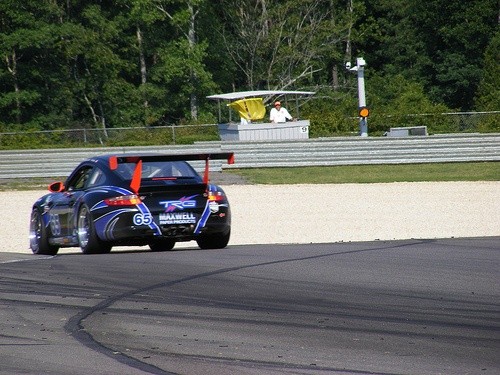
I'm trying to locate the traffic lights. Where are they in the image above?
[359,106,369,118]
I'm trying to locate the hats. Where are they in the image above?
[274,101,282,107]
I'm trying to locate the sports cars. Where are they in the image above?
[28,151,234,255]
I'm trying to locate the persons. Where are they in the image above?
[270,103,294,123]
